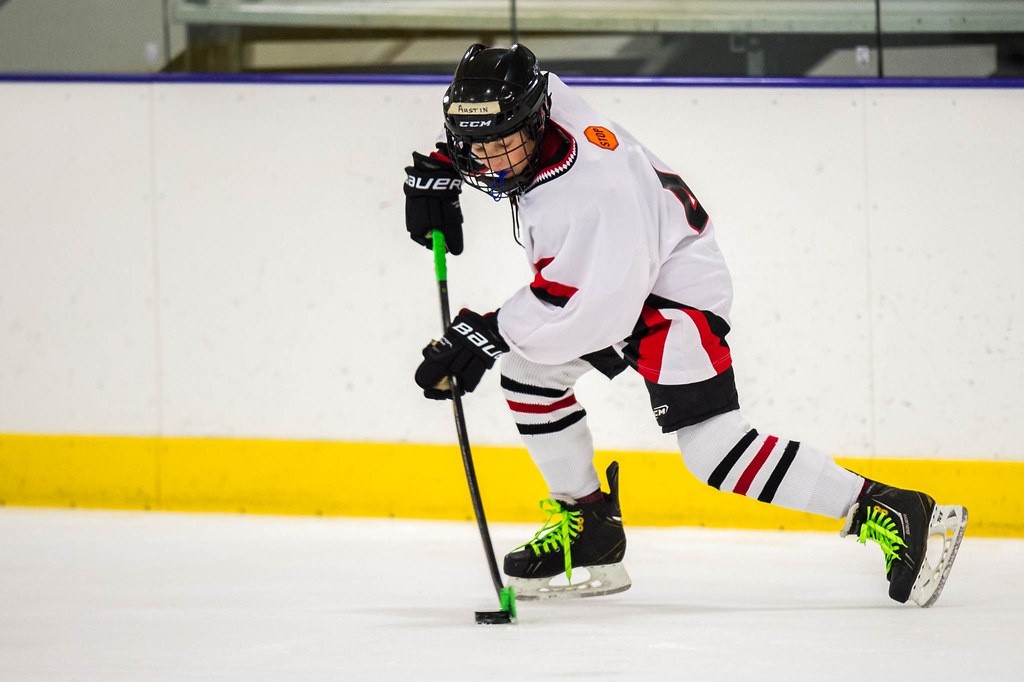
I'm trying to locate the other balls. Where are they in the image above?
[473,609,511,625]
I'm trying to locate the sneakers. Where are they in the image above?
[502,460,632,603]
[839,467,968,610]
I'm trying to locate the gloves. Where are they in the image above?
[402,150,464,256]
[414,307,511,401]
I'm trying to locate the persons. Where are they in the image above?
[402,41,969,627]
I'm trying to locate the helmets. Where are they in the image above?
[443,42,551,137]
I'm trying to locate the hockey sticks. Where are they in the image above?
[428,219,520,625]
